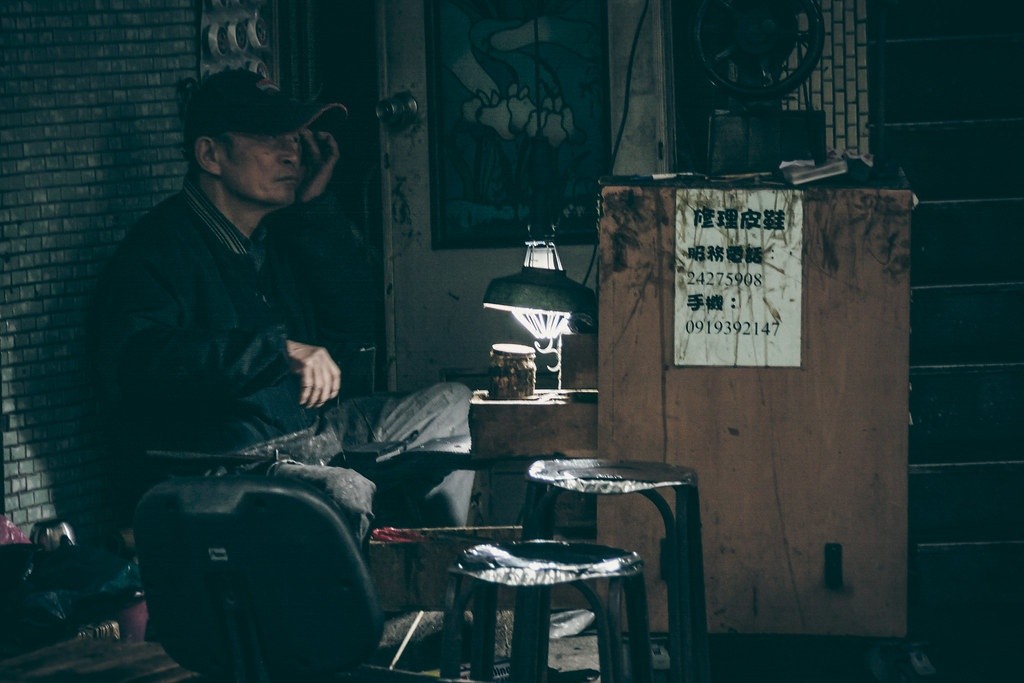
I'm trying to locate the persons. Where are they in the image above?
[91,71,596,642]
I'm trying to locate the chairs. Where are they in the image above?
[139,477,469,682]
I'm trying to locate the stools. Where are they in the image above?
[438,539,654,683]
[518,455,711,683]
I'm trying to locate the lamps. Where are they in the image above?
[480,236,597,372]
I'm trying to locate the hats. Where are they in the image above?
[185,69,349,138]
